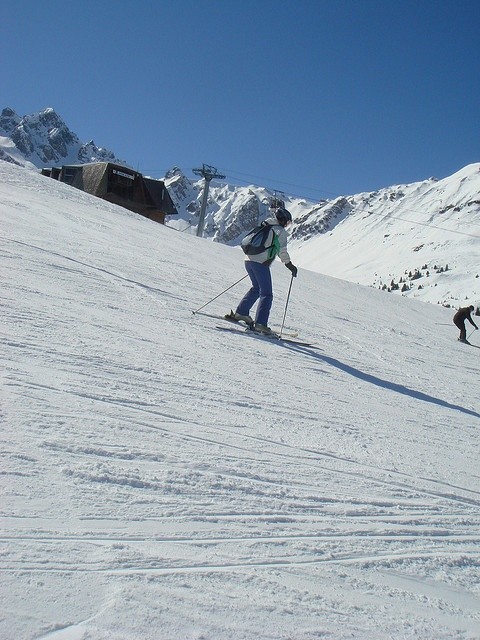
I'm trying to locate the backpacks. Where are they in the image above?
[241,221,284,254]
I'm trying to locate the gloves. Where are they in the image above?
[284,261,297,277]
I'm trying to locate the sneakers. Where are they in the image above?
[234,312,255,325]
[254,322,273,334]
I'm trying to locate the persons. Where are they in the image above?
[453,306,478,341]
[234,207,297,331]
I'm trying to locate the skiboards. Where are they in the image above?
[194,310,317,345]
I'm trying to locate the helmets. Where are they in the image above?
[276,206,292,227]
